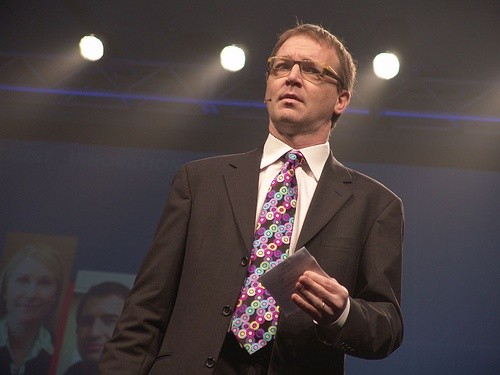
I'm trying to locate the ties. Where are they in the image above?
[231,150,305,354]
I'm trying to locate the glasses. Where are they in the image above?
[268,57,343,86]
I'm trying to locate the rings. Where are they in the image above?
[318,301,325,312]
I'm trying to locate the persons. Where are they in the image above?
[94,23,405,375]
[0,242,130,375]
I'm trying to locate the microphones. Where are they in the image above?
[264,99,272,101]
